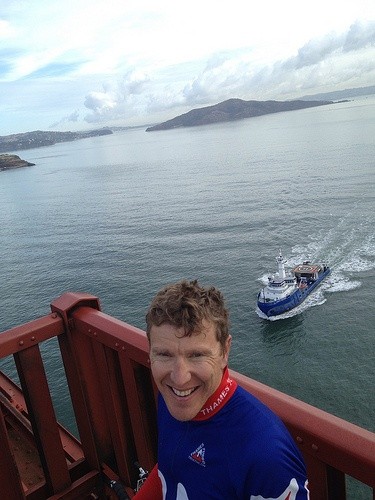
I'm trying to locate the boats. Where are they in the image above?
[257,248,330,318]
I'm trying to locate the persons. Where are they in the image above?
[130,279,311,500]
